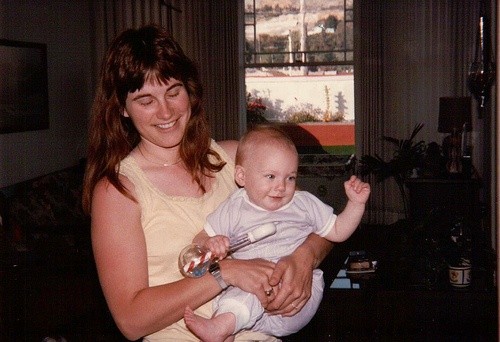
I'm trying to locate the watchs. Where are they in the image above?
[209,257,228,289]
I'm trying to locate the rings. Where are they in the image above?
[264,287,273,296]
[291,303,298,309]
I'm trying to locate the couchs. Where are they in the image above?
[0,159,125,342]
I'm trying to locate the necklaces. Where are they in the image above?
[137,145,181,166]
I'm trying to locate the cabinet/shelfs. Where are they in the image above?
[404,155,484,240]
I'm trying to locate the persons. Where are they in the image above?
[82,23,336,342]
[185,123,370,342]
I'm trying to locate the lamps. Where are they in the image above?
[437,95,473,174]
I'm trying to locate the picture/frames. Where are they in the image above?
[0,38,50,135]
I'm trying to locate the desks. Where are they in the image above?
[330,222,499,342]
